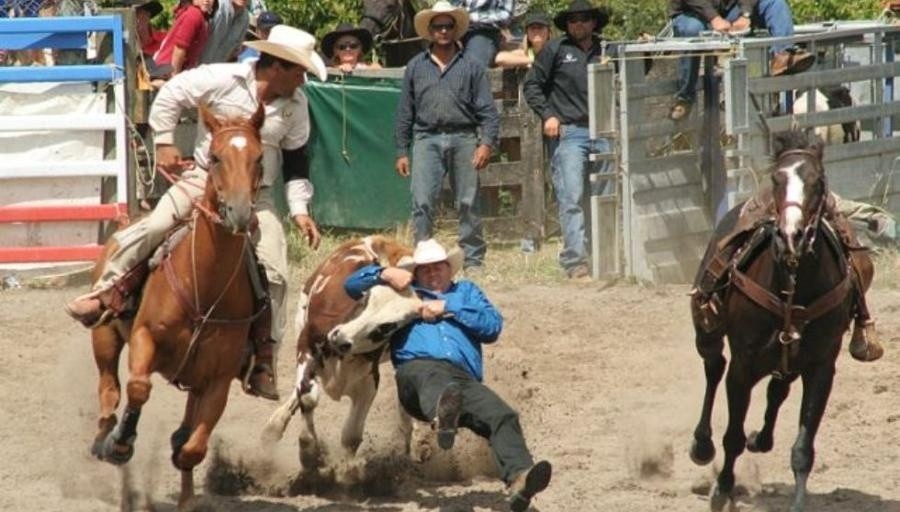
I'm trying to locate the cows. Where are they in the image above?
[260,219,460,490]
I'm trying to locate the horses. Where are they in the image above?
[87,100,268,512]
[688,122,859,512]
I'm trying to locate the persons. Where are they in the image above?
[199,1,248,65]
[663,2,819,122]
[341,236,554,512]
[442,2,523,78]
[62,22,327,401]
[0,1,65,66]
[494,12,555,216]
[524,2,657,285]
[238,10,283,62]
[134,0,219,80]
[392,1,501,276]
[321,21,383,79]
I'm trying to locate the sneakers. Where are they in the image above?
[510,460,550,511]
[437,383,461,450]
[671,100,692,121]
[770,47,815,77]
[247,364,278,402]
[63,298,112,327]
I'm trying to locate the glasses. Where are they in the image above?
[568,15,592,23]
[429,20,455,32]
[335,42,364,50]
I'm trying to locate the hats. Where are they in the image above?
[243,24,327,84]
[554,0,609,31]
[397,239,464,279]
[323,25,372,58]
[525,16,550,28]
[414,2,468,42]
[257,11,283,30]
